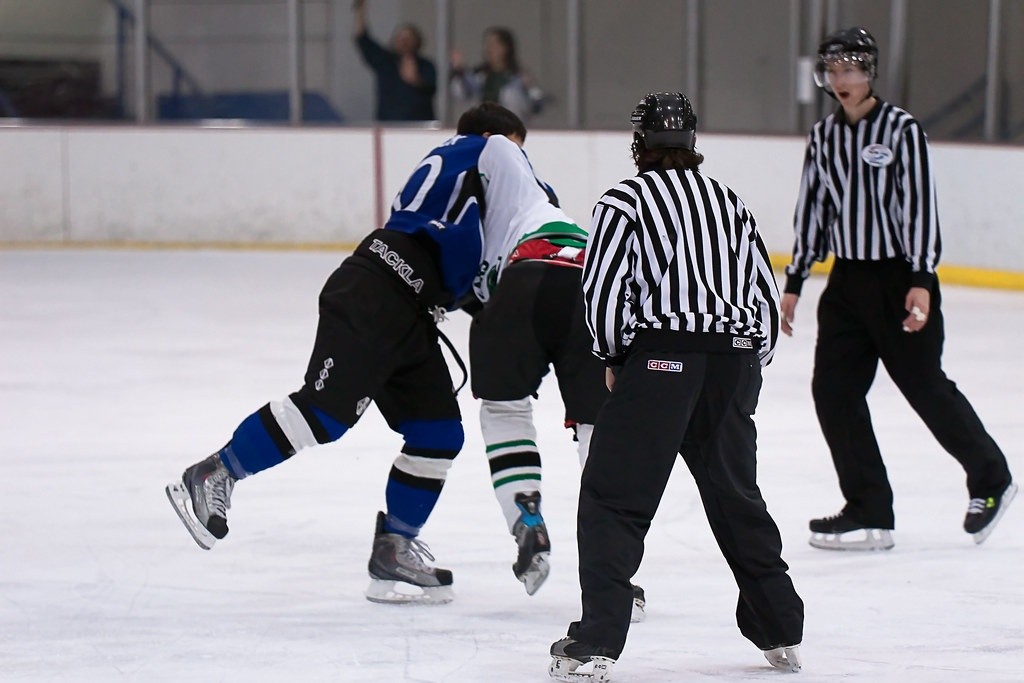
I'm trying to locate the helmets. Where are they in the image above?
[631,92,698,151]
[814,27,879,87]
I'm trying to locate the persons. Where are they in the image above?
[164,109,527,607]
[349,1,441,128]
[471,134,614,594]
[549,93,804,678]
[446,26,547,131]
[779,28,1019,549]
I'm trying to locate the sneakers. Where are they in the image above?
[631,586,652,623]
[166,452,237,551]
[548,637,617,683]
[762,641,802,673]
[512,490,551,595]
[808,511,896,551]
[963,477,1018,545]
[365,511,455,604]
